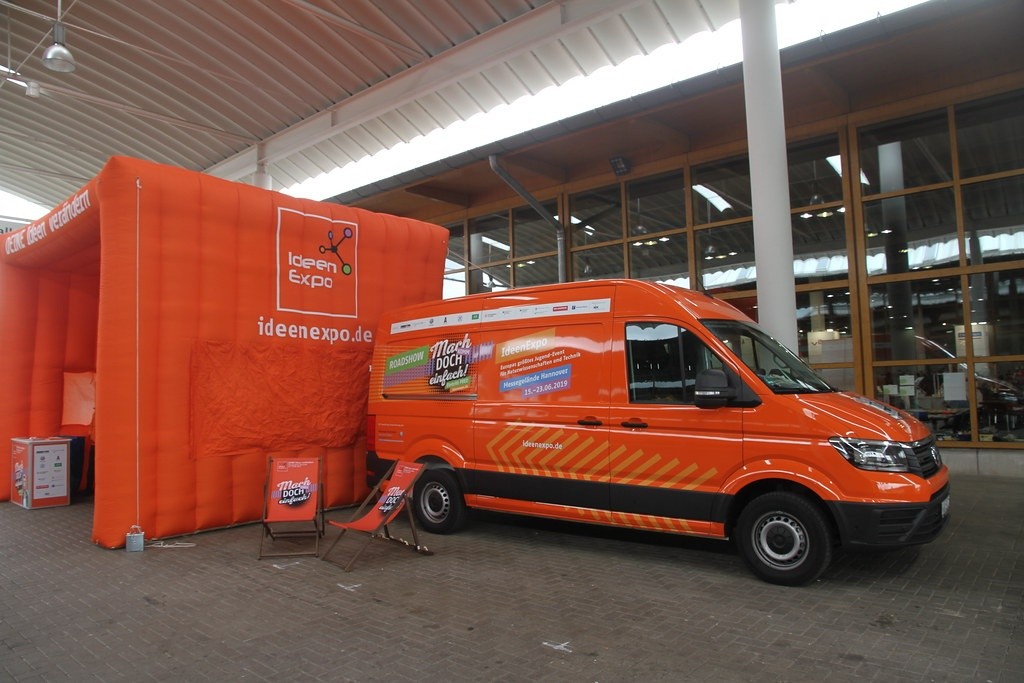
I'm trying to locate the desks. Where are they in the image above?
[979,399,1015,435]
[927,406,970,440]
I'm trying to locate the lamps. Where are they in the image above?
[483,243,496,289]
[609,156,632,177]
[800,160,838,220]
[24,81,42,99]
[583,233,594,274]
[705,227,740,261]
[41,0,78,73]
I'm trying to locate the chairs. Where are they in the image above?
[321,458,435,570]
[253,452,326,557]
[62,370,98,491]
[721,350,773,402]
[623,331,699,406]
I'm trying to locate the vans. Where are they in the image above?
[838,333,1024,440]
[364,279,950,585]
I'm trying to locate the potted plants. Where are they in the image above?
[977,430,995,442]
[941,412,972,441]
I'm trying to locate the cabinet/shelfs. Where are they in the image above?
[10,438,73,510]
